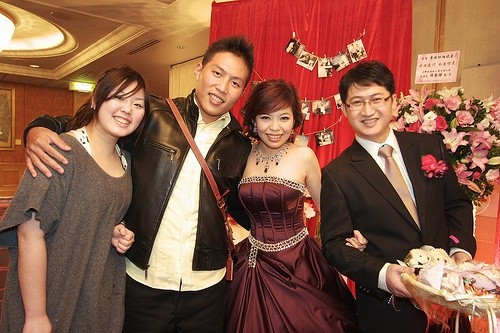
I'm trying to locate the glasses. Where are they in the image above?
[344,94,392,110]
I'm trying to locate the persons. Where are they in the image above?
[23,36,306,333]
[224,79,368,333]
[320,60,477,333]
[0,64,150,333]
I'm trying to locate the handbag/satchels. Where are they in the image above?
[225,222,235,281]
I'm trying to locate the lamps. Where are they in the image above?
[0,10,16,51]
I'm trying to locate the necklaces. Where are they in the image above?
[254,141,290,173]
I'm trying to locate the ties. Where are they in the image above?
[378,144,421,232]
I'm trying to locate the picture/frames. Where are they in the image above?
[0,87,16,150]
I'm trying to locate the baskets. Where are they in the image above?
[400,262,500,318]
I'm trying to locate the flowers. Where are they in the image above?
[421,153,449,180]
[391,83,497,206]
